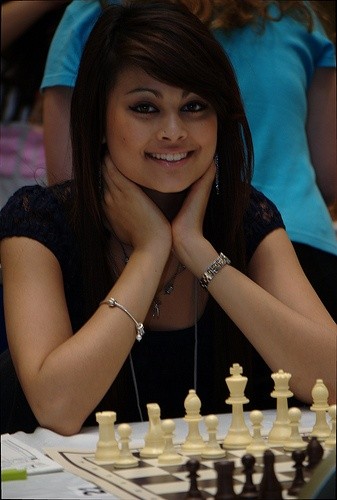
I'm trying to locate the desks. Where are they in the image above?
[1,411,330,500]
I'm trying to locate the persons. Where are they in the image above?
[0,0,337,434]
[0,0,337,321]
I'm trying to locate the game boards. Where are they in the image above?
[46,360,337,500]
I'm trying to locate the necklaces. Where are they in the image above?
[118,240,188,319]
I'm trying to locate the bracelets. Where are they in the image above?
[198,252,231,288]
[100,298,146,341]
